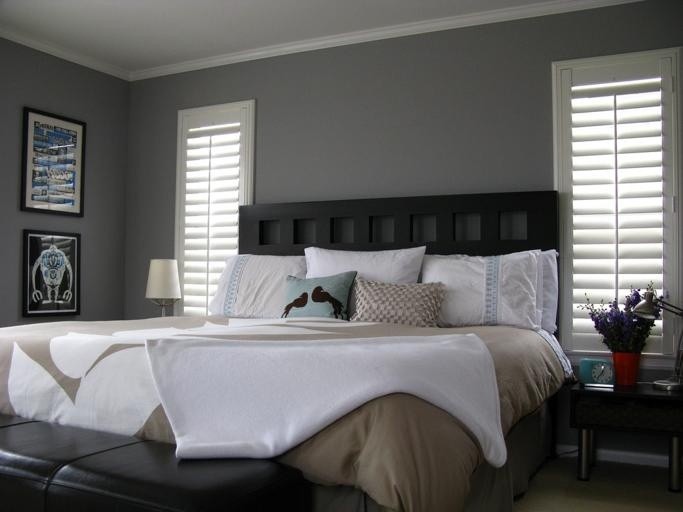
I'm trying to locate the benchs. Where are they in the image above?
[0,414,305,512]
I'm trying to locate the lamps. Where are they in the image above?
[633,292,683,391]
[145,259,182,317]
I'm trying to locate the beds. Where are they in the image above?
[0,191,558,512]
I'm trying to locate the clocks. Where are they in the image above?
[592,363,613,384]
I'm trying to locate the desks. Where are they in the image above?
[569,381,683,493]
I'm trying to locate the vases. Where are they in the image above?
[612,352,641,389]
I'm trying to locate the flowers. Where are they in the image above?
[586,282,664,353]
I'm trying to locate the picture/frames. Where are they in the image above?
[21,106,86,218]
[23,229,80,317]
